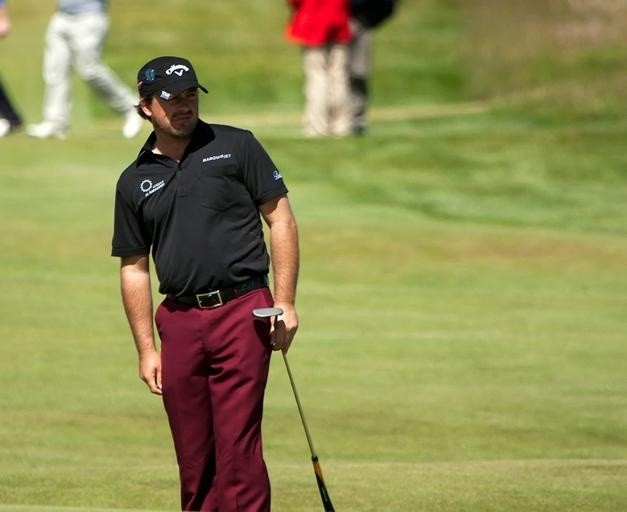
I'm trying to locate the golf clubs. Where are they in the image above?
[252,308,335,512]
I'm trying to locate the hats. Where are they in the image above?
[133,55,209,103]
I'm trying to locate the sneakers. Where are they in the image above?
[22,121,67,140]
[121,98,145,140]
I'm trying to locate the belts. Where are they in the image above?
[164,273,269,312]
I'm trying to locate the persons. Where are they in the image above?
[283,0,395,141]
[27,0,145,141]
[109,57,299,511]
[0,0,22,138]
